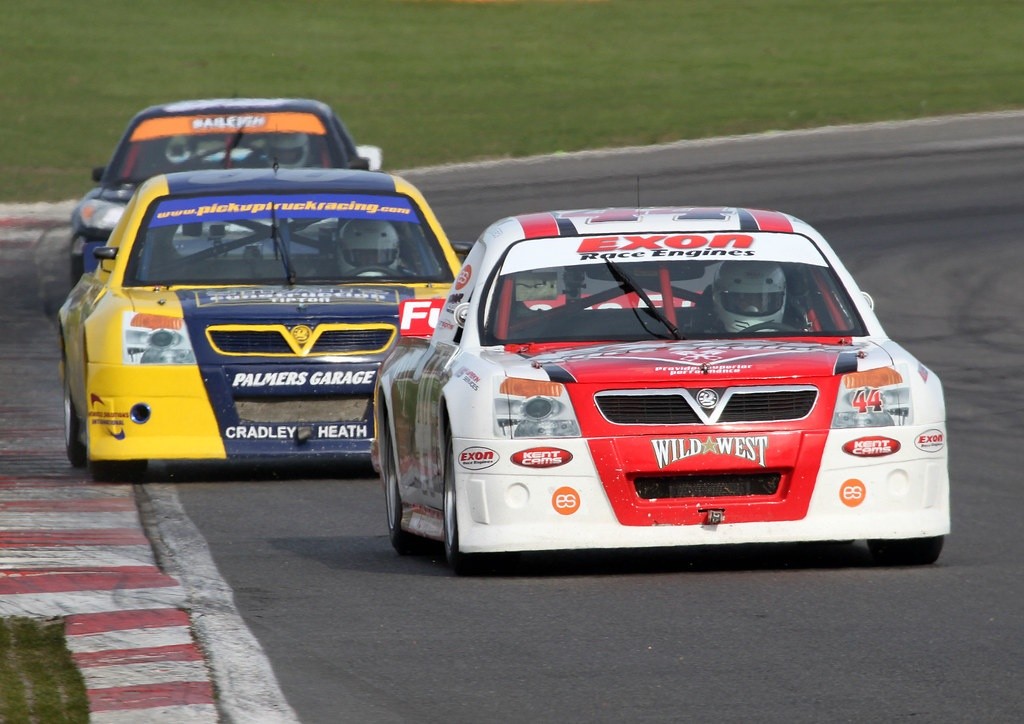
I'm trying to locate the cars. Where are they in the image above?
[57,166,471,481]
[374,206,952,577]
[70,95,370,282]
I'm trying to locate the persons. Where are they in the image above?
[336,219,417,277]
[267,134,314,169]
[709,259,788,337]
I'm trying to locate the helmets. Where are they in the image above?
[338,220,400,277]
[712,261,786,333]
[267,132,309,169]
